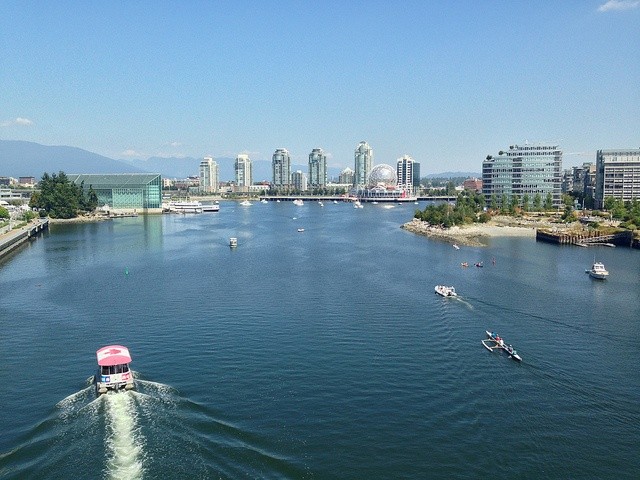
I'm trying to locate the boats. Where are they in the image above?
[582,251,609,281]
[479,329,523,363]
[434,284,456,298]
[229,238,238,248]
[92,344,137,397]
